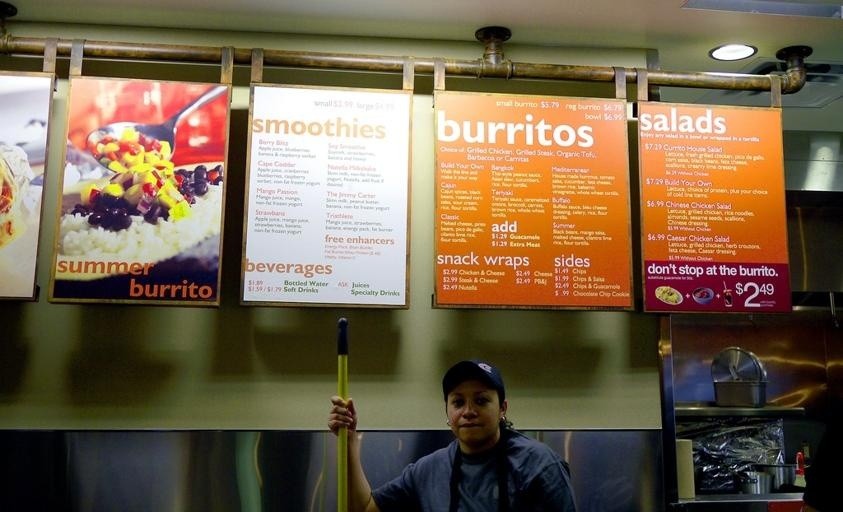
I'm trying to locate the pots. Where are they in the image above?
[714,380,768,409]
[736,459,797,496]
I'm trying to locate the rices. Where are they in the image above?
[55,181,223,280]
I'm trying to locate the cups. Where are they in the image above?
[724,290,733,306]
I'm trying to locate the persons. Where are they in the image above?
[327,359,578,509]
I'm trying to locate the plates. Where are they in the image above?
[654,286,683,305]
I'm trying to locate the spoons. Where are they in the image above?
[86,81,228,176]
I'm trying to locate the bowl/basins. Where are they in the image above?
[692,287,714,305]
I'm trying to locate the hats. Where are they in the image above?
[442,359,505,402]
